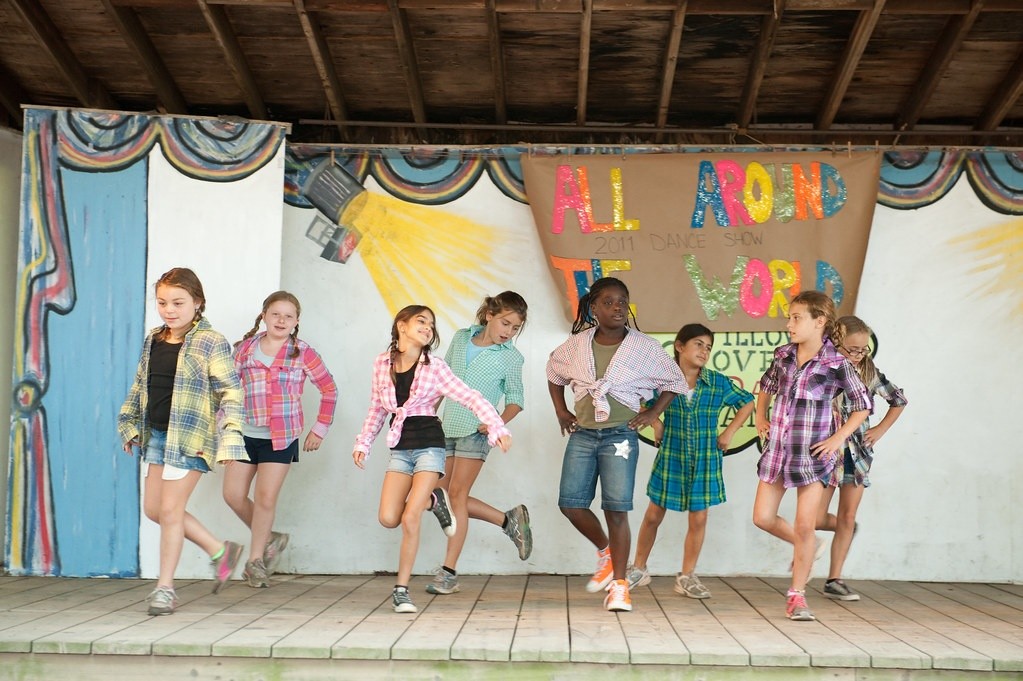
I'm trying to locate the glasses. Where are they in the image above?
[840,344,873,358]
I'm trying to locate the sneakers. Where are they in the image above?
[145,585,182,615]
[823,577,860,602]
[586,545,615,594]
[788,537,827,570]
[429,486,457,537]
[622,564,651,591]
[673,571,713,598]
[240,557,270,588]
[210,539,245,596]
[603,579,633,611]
[502,504,533,560]
[262,530,290,569]
[425,564,460,595]
[783,592,816,621]
[391,587,417,614]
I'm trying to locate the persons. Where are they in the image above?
[349,304,512,612]
[427,289,532,595]
[222,290,339,588]
[545,278,689,613]
[625,324,755,599]
[116,267,254,616]
[753,289,873,620]
[813,315,908,600]
